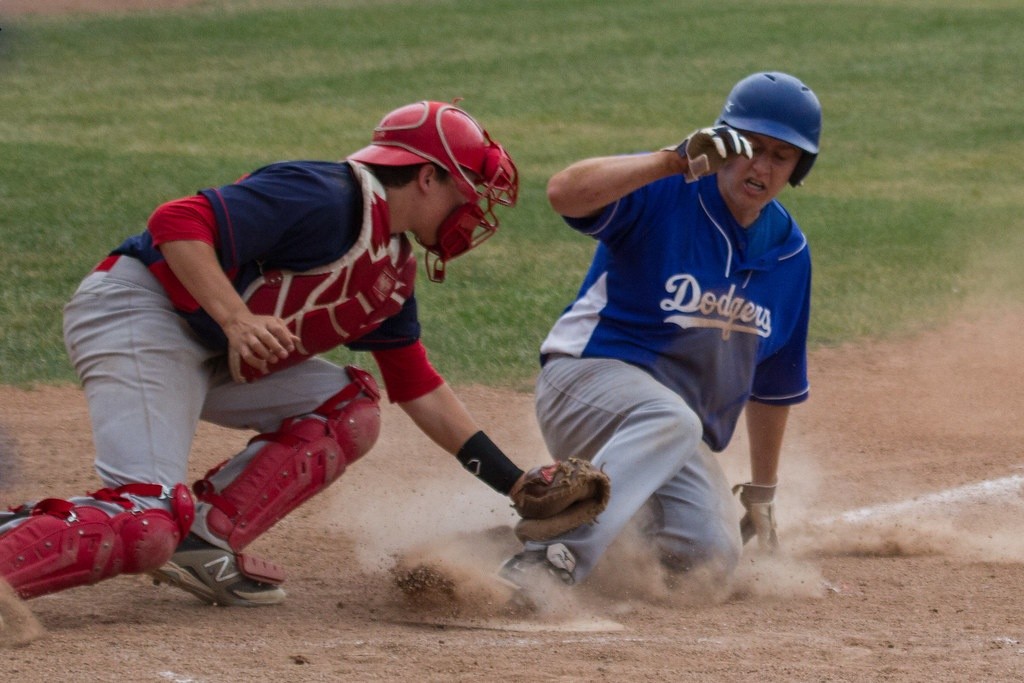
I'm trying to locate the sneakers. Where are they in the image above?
[494,542,578,597]
[144,506,286,604]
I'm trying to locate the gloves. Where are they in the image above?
[731,478,778,550]
[660,126,754,184]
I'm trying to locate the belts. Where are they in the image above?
[89,256,119,272]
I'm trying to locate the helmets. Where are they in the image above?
[346,100,487,174]
[713,71,820,187]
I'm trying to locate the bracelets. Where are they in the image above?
[457,431,525,499]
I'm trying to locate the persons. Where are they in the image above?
[1,99,610,603]
[491,71,825,592]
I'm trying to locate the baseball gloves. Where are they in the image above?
[510,456,613,544]
